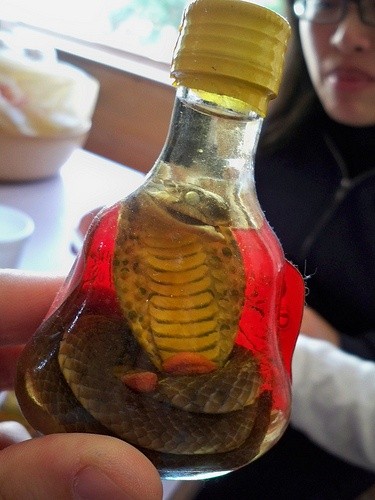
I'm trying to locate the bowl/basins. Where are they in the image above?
[0,123,92,183]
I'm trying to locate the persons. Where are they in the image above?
[79,0,375,364]
[0,269,375,500]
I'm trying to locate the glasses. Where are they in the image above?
[292,0,375,27]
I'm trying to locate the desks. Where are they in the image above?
[0,149,204,500]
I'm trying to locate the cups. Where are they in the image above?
[0,206,35,270]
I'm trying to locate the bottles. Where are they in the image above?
[14,0,307,478]
